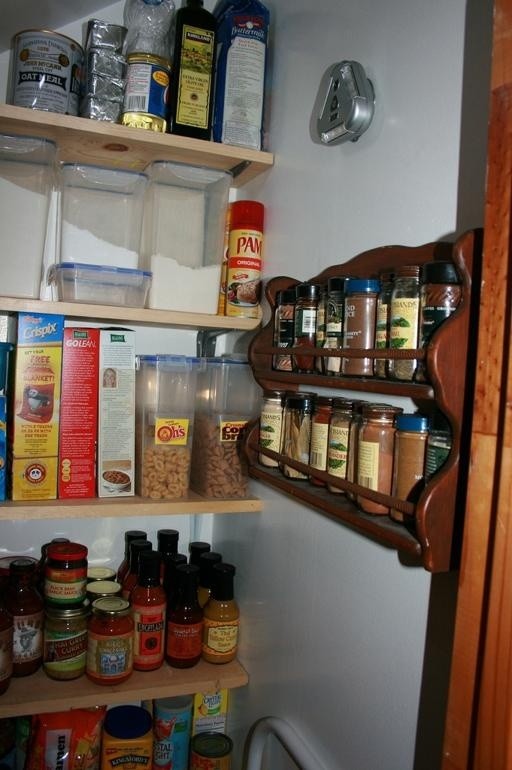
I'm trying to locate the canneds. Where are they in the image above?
[188,732,233,770]
[261,387,452,516]
[272,265,461,382]
[0,538,134,686]
[119,53,172,135]
[8,29,83,116]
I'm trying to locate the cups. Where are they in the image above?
[134,353,257,502]
[60,158,150,317]
[143,156,226,312]
[0,128,56,300]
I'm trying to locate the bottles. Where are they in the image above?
[261,386,459,523]
[0,559,46,693]
[120,528,241,695]
[123,0,270,152]
[215,198,267,318]
[268,259,460,381]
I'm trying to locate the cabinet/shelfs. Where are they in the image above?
[1,94,263,720]
[240,232,487,572]
